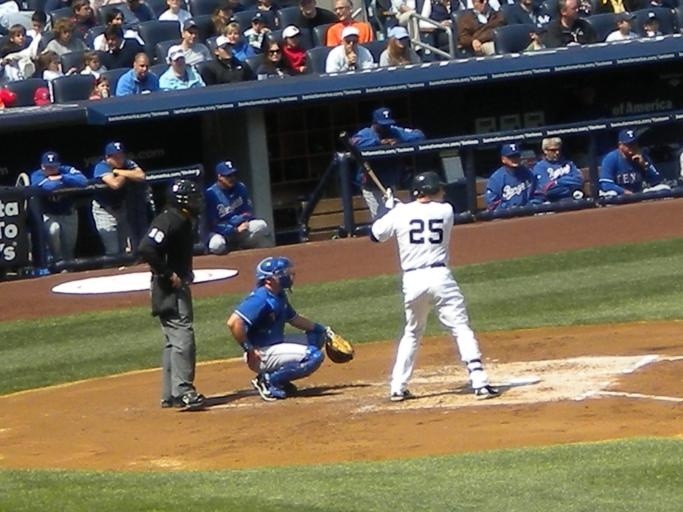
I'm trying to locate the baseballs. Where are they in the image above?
[573,190,584,200]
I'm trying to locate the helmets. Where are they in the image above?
[170,179,205,217]
[408,171,448,197]
[256,257,296,293]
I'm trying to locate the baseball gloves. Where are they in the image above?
[326,325,354,363]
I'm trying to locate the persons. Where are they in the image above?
[204,160,268,255]
[1,1,683,108]
[367,170,500,402]
[92,142,145,269]
[137,179,205,411]
[226,256,335,402]
[349,109,426,237]
[484,141,538,212]
[598,129,662,204]
[31,152,89,274]
[529,137,585,205]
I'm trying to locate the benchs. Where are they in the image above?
[293,161,608,245]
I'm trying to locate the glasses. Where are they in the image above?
[170,50,183,56]
[270,48,281,56]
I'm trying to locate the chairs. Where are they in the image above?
[0,0,683,114]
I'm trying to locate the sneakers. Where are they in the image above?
[390,387,414,401]
[160,391,205,412]
[474,386,500,400]
[251,373,278,401]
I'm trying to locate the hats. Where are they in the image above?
[105,141,126,155]
[214,35,236,49]
[616,128,640,144]
[34,88,50,106]
[387,26,410,40]
[500,143,523,158]
[251,11,265,23]
[614,11,658,24]
[41,151,61,167]
[216,160,239,177]
[283,25,300,40]
[168,45,185,62]
[372,107,396,126]
[181,18,198,31]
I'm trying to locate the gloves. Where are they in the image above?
[381,187,402,209]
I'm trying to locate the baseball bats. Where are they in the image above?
[340,132,386,194]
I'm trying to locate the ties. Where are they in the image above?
[342,26,360,39]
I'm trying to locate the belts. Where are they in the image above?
[404,262,445,272]
